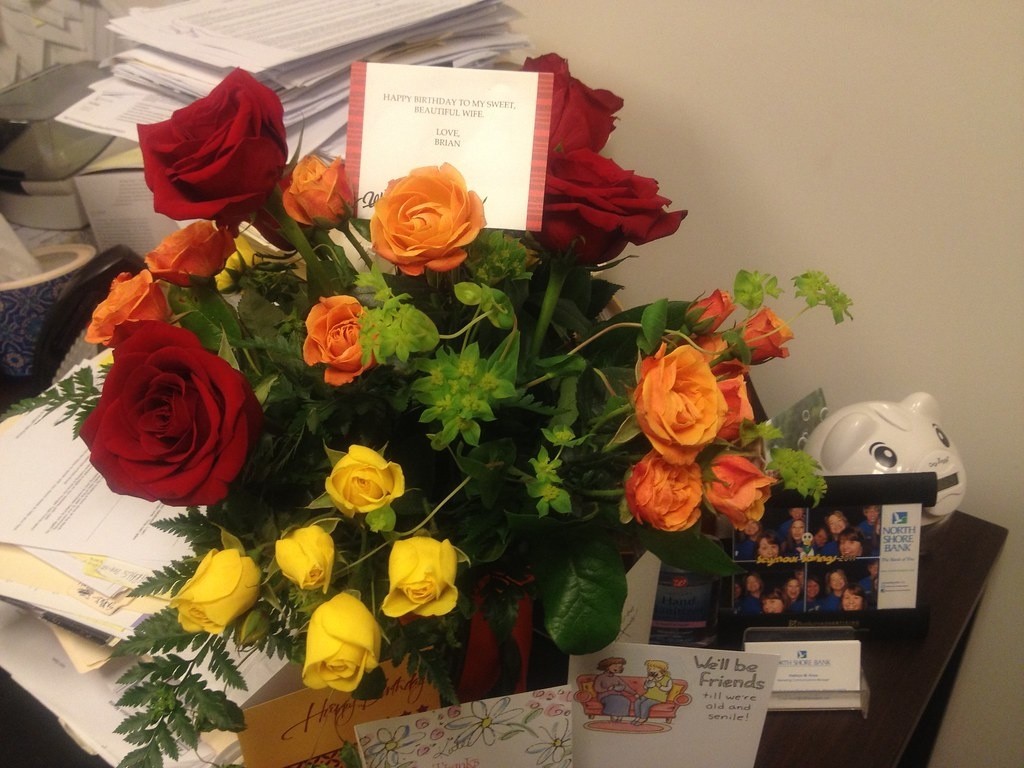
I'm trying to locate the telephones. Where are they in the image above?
[32,243,150,389]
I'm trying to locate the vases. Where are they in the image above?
[369,563,535,707]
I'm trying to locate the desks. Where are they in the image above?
[0,240,1012,768]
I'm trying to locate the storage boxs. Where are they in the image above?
[73,137,193,260]
[234,644,441,768]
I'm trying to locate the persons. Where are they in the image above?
[733,504,881,612]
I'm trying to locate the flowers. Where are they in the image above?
[0,51,861,768]
